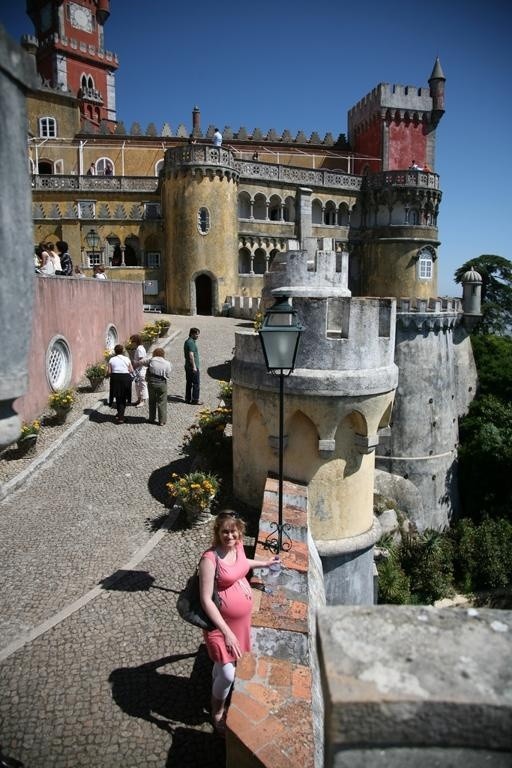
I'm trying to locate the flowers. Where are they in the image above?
[155,317,171,327]
[164,471,221,509]
[19,419,43,436]
[141,321,161,341]
[218,380,233,397]
[103,348,114,360]
[84,361,108,378]
[194,405,231,428]
[177,424,210,460]
[48,388,78,410]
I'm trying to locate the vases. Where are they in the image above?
[219,397,233,406]
[88,375,107,392]
[160,325,170,338]
[181,496,215,526]
[189,454,215,474]
[52,407,76,425]
[16,435,38,460]
[206,425,226,438]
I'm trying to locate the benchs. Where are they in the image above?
[143,303,162,314]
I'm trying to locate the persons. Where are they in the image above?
[188,133,196,144]
[138,348,171,426]
[130,334,148,408]
[184,328,204,405]
[107,344,133,424]
[105,163,113,176]
[198,516,284,739]
[33,240,107,281]
[90,163,95,176]
[212,129,222,147]
[408,159,432,172]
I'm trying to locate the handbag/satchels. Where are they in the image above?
[177,576,220,632]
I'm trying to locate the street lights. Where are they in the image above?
[85,229,101,278]
[258,292,309,553]
